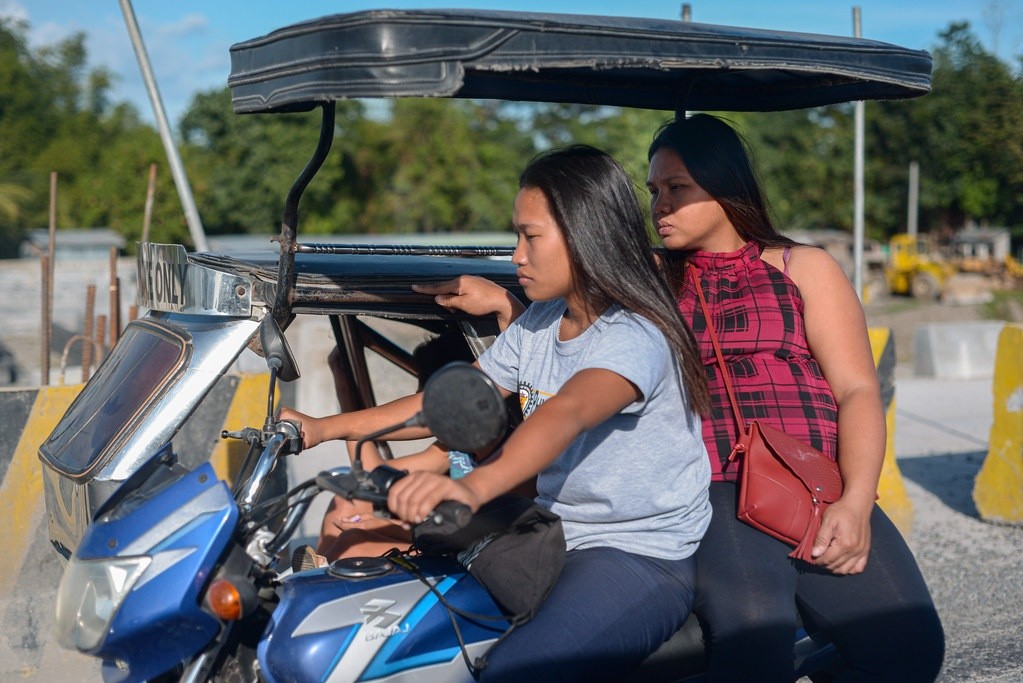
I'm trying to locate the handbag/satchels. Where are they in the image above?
[736,420,880,565]
[380,487,568,673]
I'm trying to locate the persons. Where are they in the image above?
[410,113,946,683]
[290,275,528,572]
[278,145,715,683]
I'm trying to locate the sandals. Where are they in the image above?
[291,544,330,573]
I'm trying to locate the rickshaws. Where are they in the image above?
[37,6,940,681]
